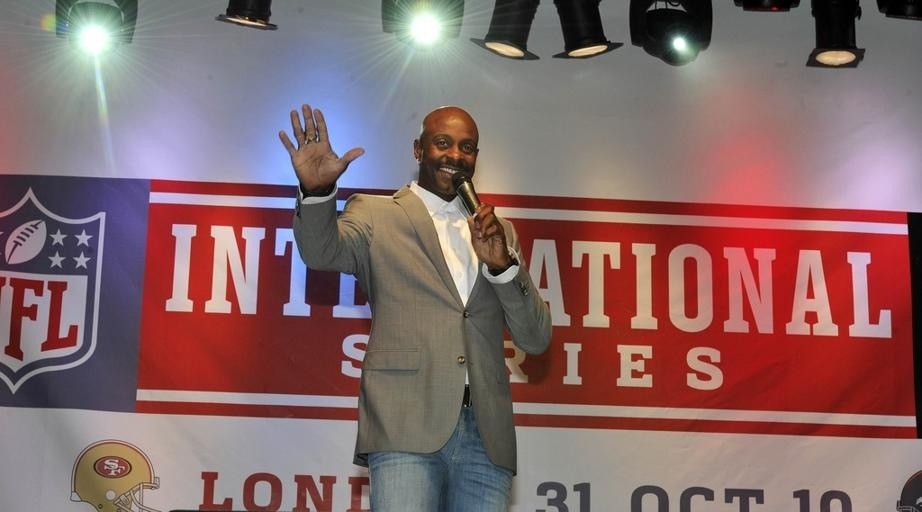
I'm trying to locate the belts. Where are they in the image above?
[462,384,472,406]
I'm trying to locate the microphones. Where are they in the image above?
[452,172,480,217]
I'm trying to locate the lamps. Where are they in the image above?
[55,0,137,45]
[381,0,464,49]
[469,0,542,60]
[628,1,711,66]
[550,0,623,59]
[805,0,865,67]
[216,0,278,30]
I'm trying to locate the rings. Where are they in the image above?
[305,131,317,141]
[297,134,304,138]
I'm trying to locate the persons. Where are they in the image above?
[280,103,552,512]
